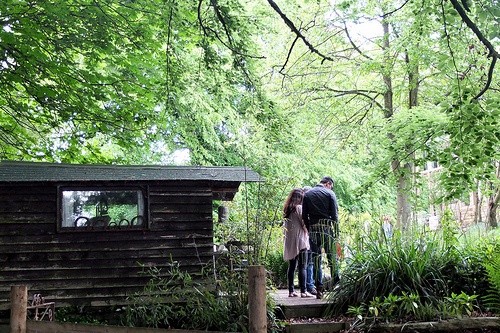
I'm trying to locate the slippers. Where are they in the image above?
[302,293,313,298]
[289,293,298,297]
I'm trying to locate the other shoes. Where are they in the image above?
[310,287,324,299]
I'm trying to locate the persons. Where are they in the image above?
[382,217,393,236]
[344,239,357,264]
[301,185,317,297]
[301,176,341,300]
[415,233,429,251]
[281,188,314,298]
[335,242,343,268]
